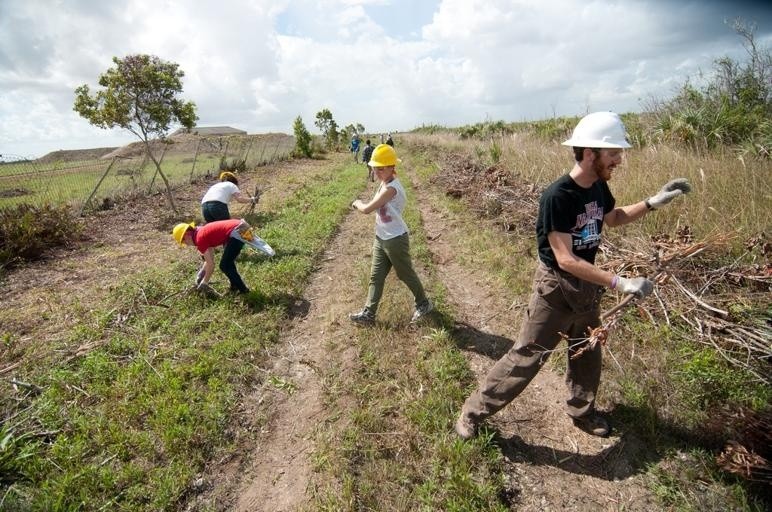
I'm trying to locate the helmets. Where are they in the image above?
[173,222,195,247]
[220,172,236,180]
[368,144,402,167]
[560,112,633,148]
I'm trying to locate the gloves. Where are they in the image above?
[196,270,208,293]
[612,274,654,299]
[649,178,692,208]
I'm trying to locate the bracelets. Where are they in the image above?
[644,198,657,212]
[252,196,257,204]
[197,269,206,279]
[611,275,617,289]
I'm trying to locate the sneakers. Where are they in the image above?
[456,412,478,439]
[412,300,433,321]
[577,414,609,435]
[349,312,375,322]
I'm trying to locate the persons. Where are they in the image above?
[347,133,393,182]
[201,172,259,225]
[456,111,691,444]
[173,219,254,294]
[349,144,433,325]
